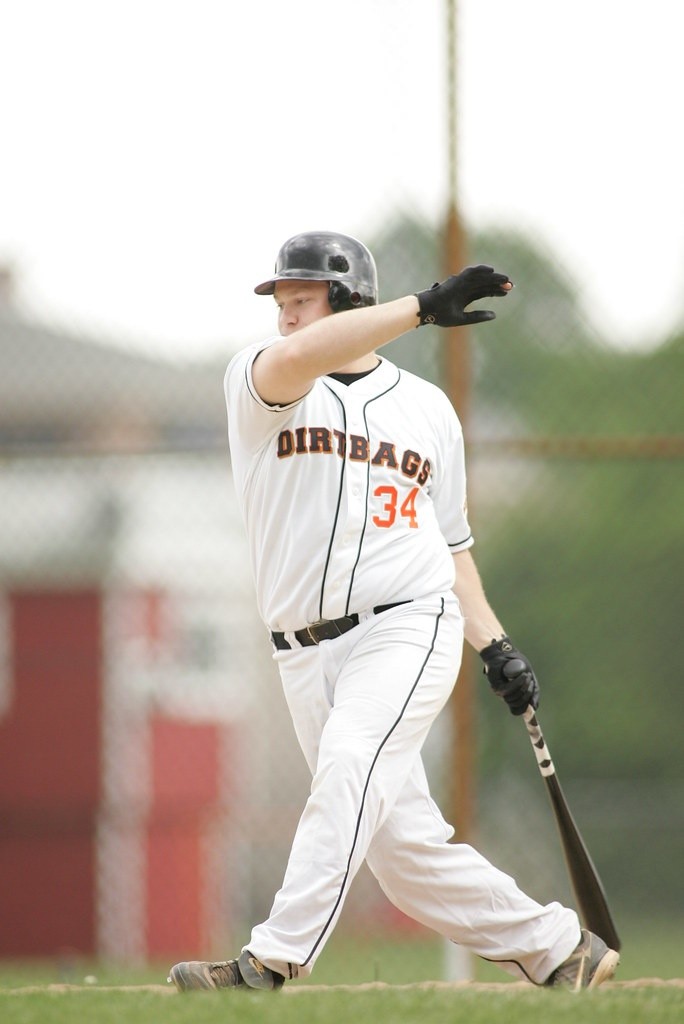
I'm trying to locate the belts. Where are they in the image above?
[272,600,412,650]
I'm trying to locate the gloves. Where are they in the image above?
[415,264,514,328]
[480,634,539,715]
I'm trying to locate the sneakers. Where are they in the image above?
[545,929,620,996]
[170,950,285,995]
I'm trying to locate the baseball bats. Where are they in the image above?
[514,695,624,958]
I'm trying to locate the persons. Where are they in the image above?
[167,233,619,992]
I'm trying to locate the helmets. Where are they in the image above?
[254,231,378,314]
